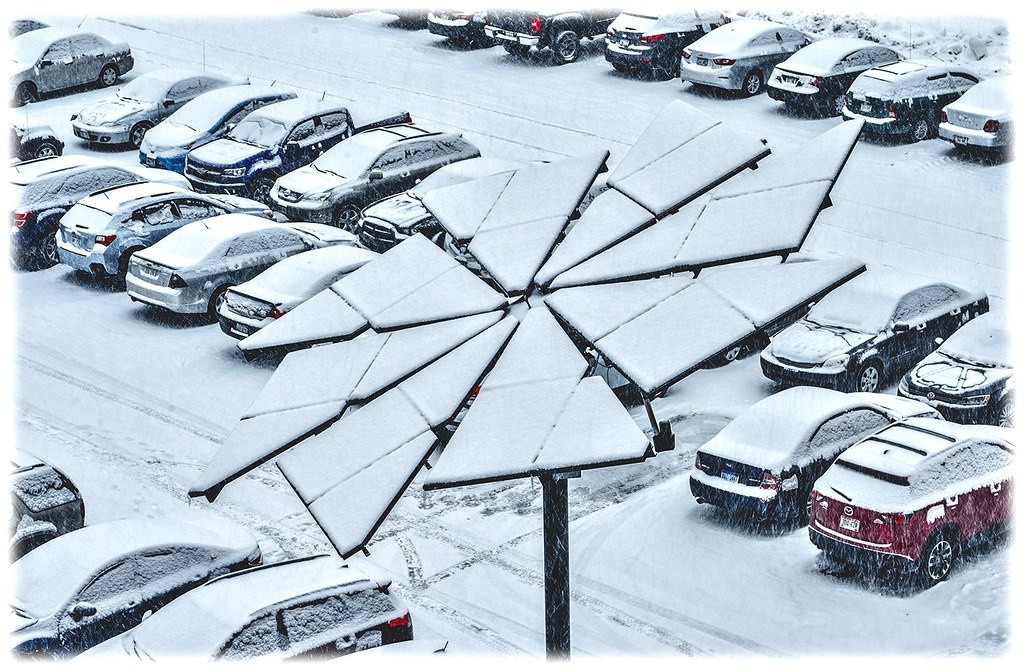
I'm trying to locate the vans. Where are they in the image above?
[67,552,415,664]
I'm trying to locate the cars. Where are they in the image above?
[69,64,251,149]
[679,18,825,97]
[269,121,557,295]
[938,73,1014,163]
[11,511,264,663]
[125,212,359,324]
[10,447,85,566]
[138,83,301,176]
[766,38,909,118]
[298,7,623,66]
[689,384,948,530]
[759,267,990,394]
[217,243,383,360]
[10,119,64,163]
[9,24,136,108]
[9,153,196,274]
[896,310,1018,428]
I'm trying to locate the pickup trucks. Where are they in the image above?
[183,93,414,211]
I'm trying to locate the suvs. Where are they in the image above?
[602,8,731,81]
[52,178,279,292]
[841,59,986,144]
[806,417,1015,590]
